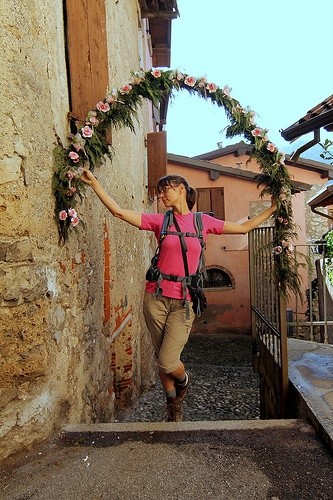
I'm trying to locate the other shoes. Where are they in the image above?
[175,370,192,401]
[167,398,183,422]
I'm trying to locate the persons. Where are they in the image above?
[79,167,279,421]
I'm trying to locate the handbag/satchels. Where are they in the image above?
[188,285,208,315]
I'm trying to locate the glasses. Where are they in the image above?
[157,181,184,193]
